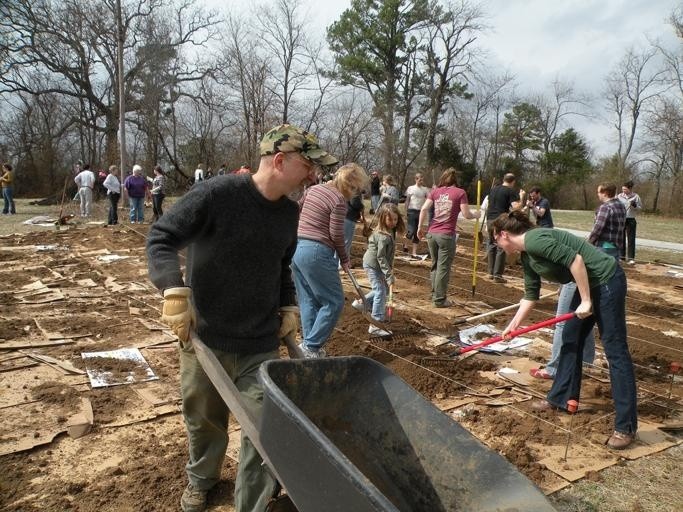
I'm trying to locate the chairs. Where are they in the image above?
[351,300,363,312]
[435,301,450,307]
[531,400,556,410]
[410,255,422,260]
[298,342,318,359]
[368,325,393,336]
[493,276,507,283]
[606,430,633,449]
[629,259,635,265]
[403,243,408,253]
[529,368,553,379]
[180,483,208,512]
[317,348,326,358]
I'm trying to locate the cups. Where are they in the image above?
[493,235,500,245]
[285,152,321,177]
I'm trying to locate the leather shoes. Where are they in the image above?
[161,286,196,342]
[631,201,636,208]
[276,306,298,353]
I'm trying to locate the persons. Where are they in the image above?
[0,163,17,216]
[101,164,121,226]
[333,195,365,270]
[616,180,641,263]
[194,160,250,184]
[291,162,368,360]
[417,167,481,308]
[528,277,596,380]
[144,165,166,220]
[487,173,526,282]
[144,123,340,512]
[403,172,432,260]
[351,203,406,337]
[377,174,400,241]
[529,187,553,228]
[488,208,638,449]
[123,163,147,225]
[586,182,627,262]
[370,171,380,213]
[74,163,96,217]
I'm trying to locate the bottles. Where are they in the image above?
[259,124,338,166]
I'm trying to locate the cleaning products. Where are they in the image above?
[178,313,558,511]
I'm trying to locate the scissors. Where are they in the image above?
[477,177,496,244]
[360,192,372,237]
[347,266,393,336]
